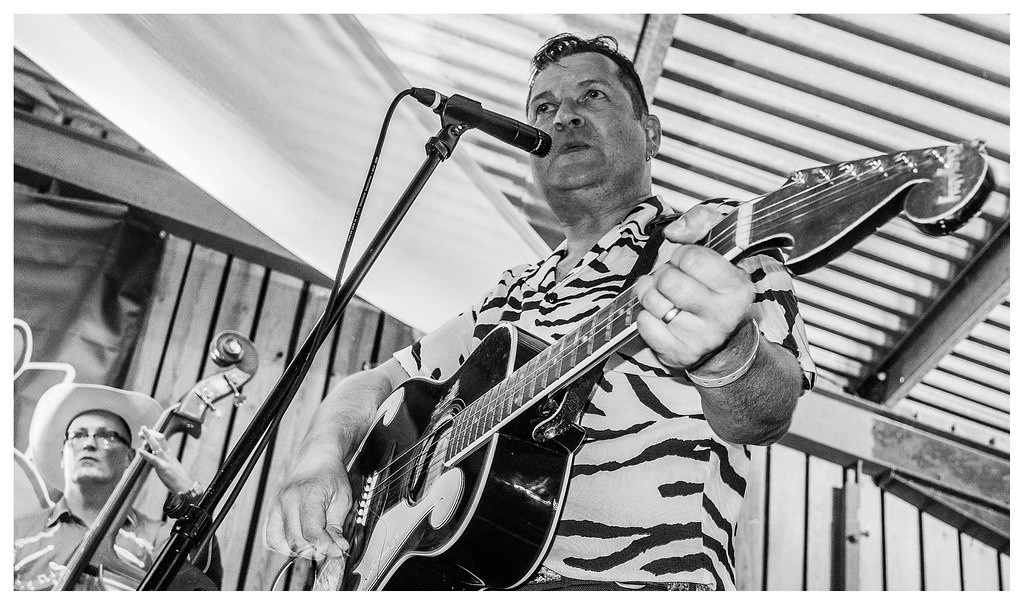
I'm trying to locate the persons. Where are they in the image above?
[264,32,818,591]
[12,383,223,591]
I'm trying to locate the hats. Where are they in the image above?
[29,383,166,511]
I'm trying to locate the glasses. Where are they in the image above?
[64,430,131,449]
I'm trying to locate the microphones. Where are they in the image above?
[411,88,552,158]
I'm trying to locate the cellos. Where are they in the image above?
[50,328,257,590]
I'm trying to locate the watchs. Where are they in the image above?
[182,481,203,501]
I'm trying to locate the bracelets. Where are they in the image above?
[684,316,760,388]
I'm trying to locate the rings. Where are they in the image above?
[152,448,162,455]
[662,305,682,324]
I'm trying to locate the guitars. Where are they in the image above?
[266,137,995,590]
